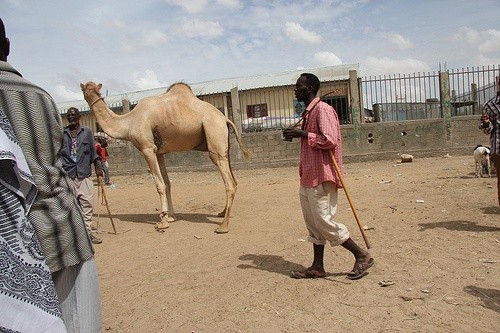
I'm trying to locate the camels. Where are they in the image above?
[79,80,252,233]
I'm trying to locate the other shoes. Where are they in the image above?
[89,234,102,244]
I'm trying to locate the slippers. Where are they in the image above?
[346,258,374,279]
[291,268,323,279]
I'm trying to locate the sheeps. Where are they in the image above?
[472,144,492,178]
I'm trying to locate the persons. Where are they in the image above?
[478,78,500,207]
[281,73,375,280]
[61,107,112,244]
[0,17,103,333]
